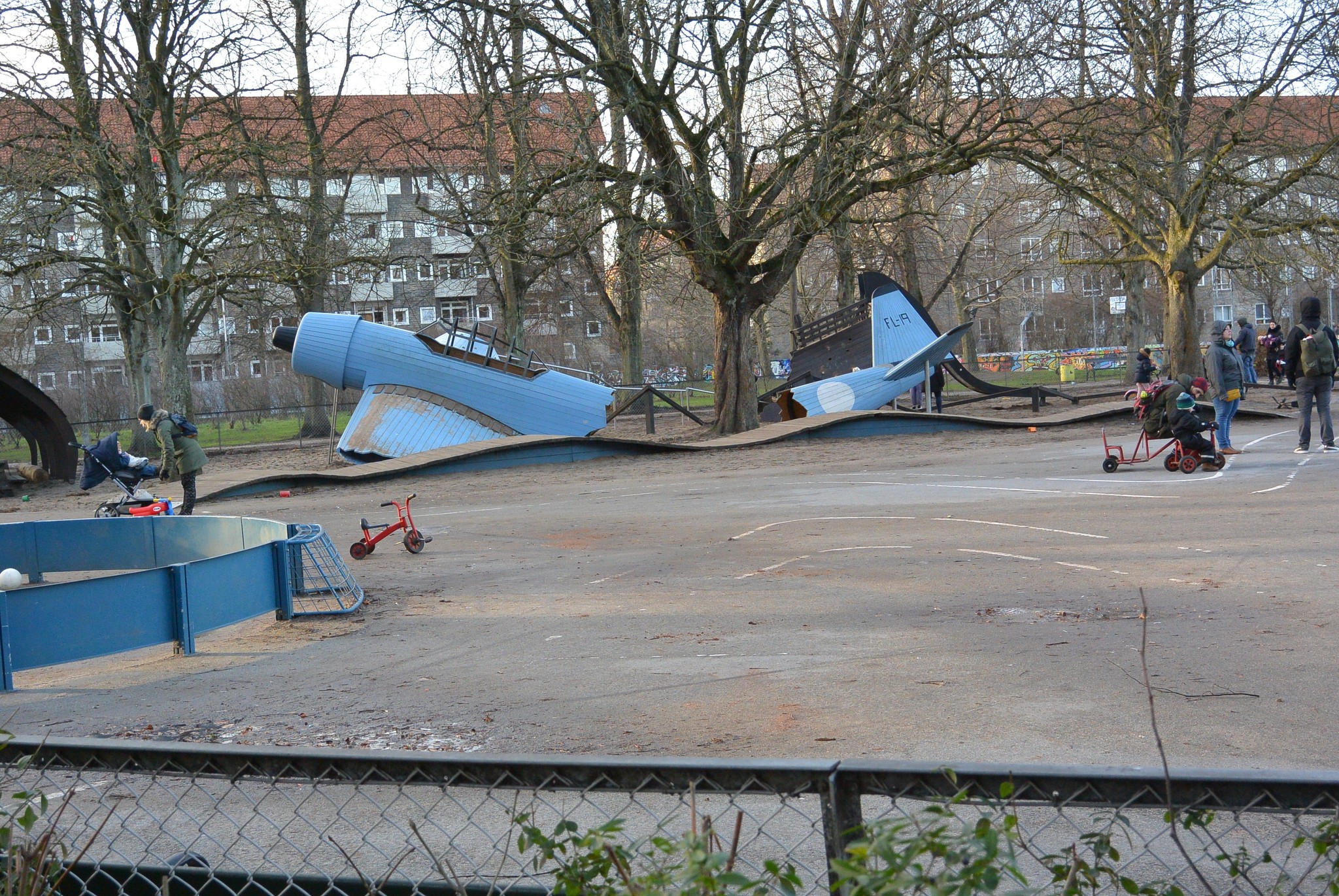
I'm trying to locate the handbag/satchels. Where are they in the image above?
[1222,388,1241,401]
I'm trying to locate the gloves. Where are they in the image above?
[1330,375,1336,390]
[158,469,169,481]
[1287,377,1297,391]
[1204,420,1221,432]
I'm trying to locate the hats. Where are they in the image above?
[1191,376,1208,396]
[1176,392,1196,410]
[136,404,155,420]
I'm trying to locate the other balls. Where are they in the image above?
[0,568,22,591]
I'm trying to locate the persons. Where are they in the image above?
[1169,392,1219,471]
[1257,335,1287,352]
[1135,347,1159,399]
[1203,320,1248,454]
[1332,319,1339,347]
[1143,372,1209,459]
[921,363,945,414]
[909,383,923,409]
[1260,320,1284,385]
[1281,296,1339,455]
[1276,340,1287,380]
[117,440,162,479]
[1233,317,1259,389]
[136,403,209,515]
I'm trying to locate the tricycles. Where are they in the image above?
[1099,390,1226,474]
[349,492,435,561]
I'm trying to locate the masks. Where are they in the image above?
[1224,338,1235,348]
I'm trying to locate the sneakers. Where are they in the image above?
[1276,376,1283,384]
[1200,460,1220,472]
[1266,380,1274,386]
[1322,443,1339,454]
[1292,446,1309,454]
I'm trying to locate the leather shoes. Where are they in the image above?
[1219,446,1241,456]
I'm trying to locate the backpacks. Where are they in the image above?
[1293,321,1338,377]
[154,412,197,440]
[1131,379,1177,421]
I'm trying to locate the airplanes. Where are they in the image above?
[1241,372,1339,410]
[266,267,977,465]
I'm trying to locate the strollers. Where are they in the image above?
[68,432,177,519]
